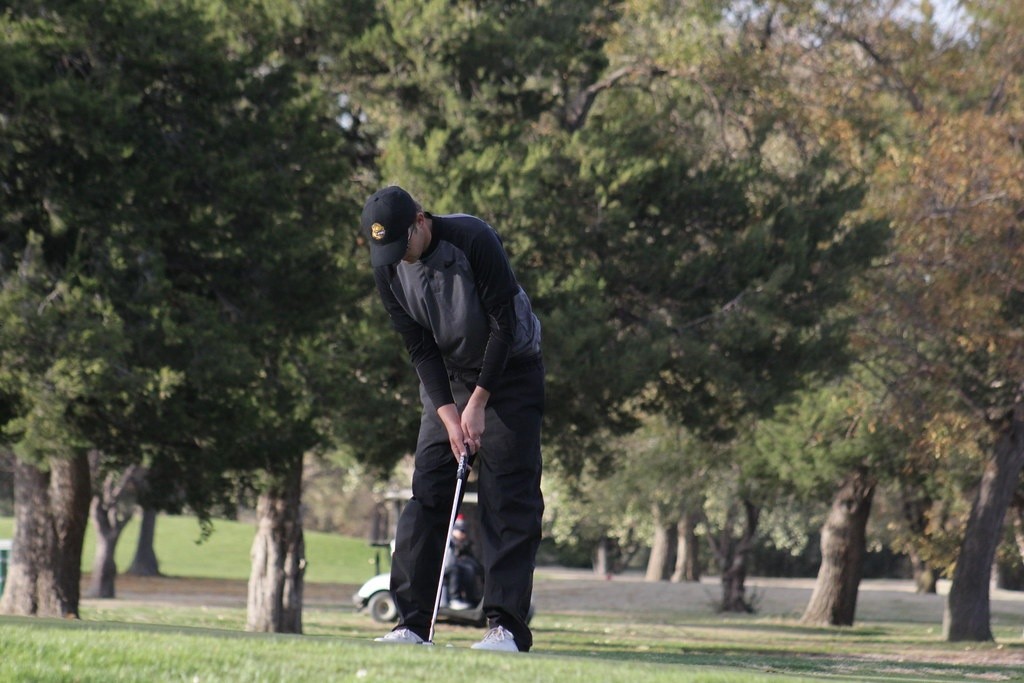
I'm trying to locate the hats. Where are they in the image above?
[453,514,466,532]
[358,184,417,267]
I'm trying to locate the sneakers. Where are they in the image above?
[374,629,423,644]
[470,625,519,651]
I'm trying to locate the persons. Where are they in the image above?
[444,513,484,611]
[360,183,546,651]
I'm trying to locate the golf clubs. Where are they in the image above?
[416,443,470,644]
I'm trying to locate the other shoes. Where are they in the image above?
[448,598,472,610]
